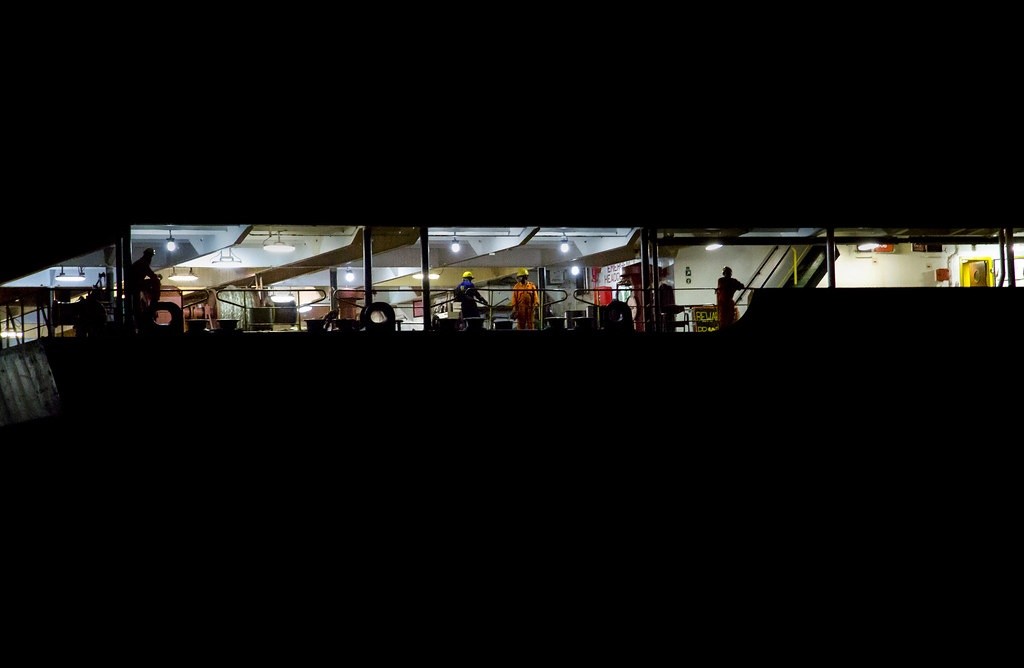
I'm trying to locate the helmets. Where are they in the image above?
[517,268,529,277]
[462,271,474,279]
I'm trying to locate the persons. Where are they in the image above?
[130,247,160,322]
[717,267,745,329]
[453,271,488,330]
[510,268,540,330]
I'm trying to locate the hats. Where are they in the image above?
[723,266,732,272]
[143,247,156,254]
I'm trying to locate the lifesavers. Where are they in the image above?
[359,301,396,332]
[602,301,632,332]
[142,301,183,335]
